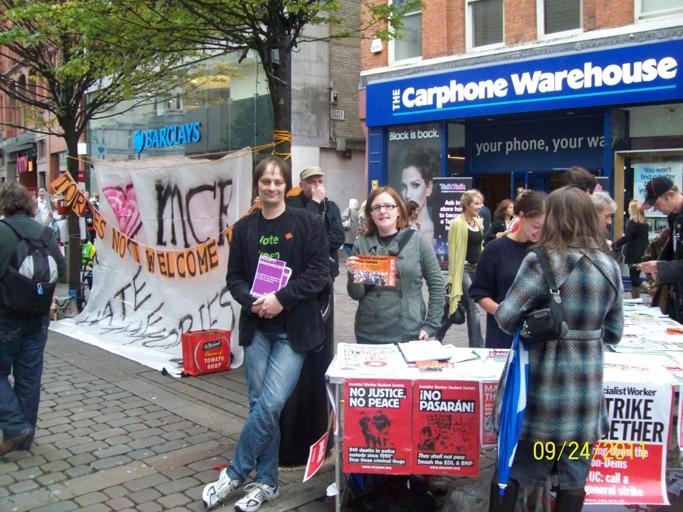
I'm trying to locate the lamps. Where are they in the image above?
[370,38,383,56]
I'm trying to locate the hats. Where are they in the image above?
[639,177,674,210]
[300,167,325,180]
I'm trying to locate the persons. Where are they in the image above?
[0,177,64,453]
[396,151,436,232]
[287,166,343,278]
[31,186,99,245]
[335,185,648,351]
[344,187,451,495]
[200,155,331,512]
[633,176,683,324]
[561,165,598,194]
[485,185,624,509]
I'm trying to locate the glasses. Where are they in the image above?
[369,200,399,212]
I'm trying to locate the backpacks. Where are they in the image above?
[1,236,56,318]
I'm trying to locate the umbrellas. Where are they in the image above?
[496,330,531,496]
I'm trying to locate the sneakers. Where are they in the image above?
[0,428,34,455]
[233,480,281,512]
[202,466,243,509]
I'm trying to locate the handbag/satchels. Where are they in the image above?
[517,305,569,353]
